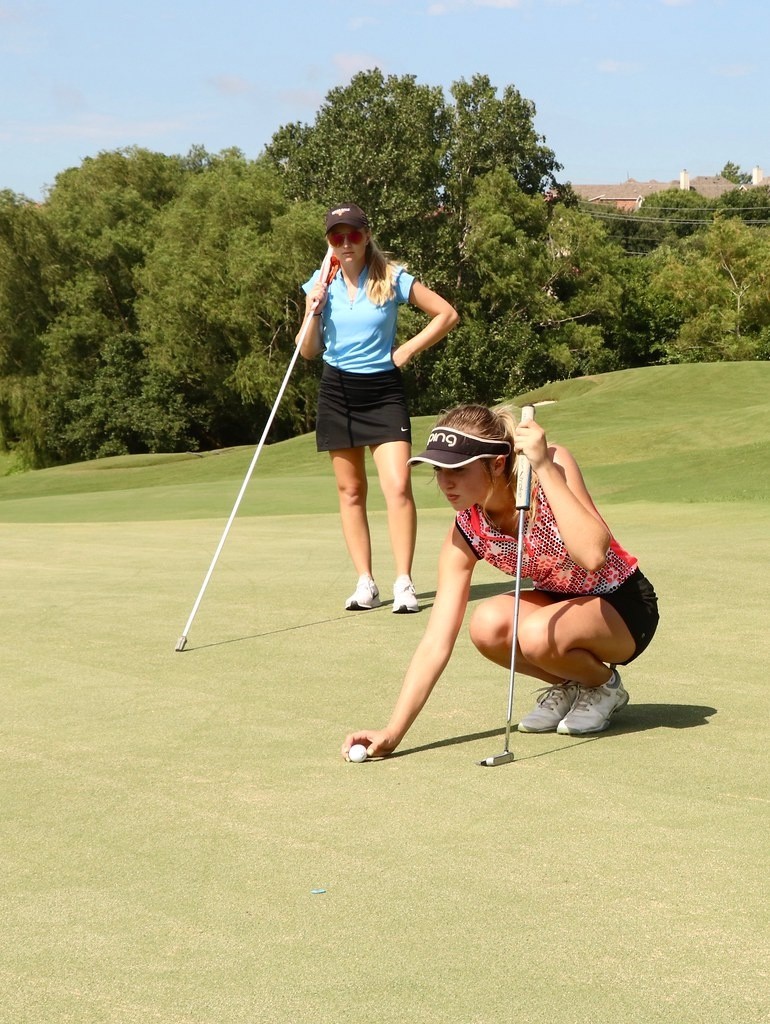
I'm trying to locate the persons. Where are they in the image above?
[341,405,659,763]
[294,203,458,612]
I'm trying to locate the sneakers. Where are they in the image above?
[392,583,420,612]
[556,669,630,735]
[346,579,382,609]
[517,679,580,733]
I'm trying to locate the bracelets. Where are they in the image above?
[314,311,322,316]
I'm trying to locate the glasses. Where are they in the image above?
[328,232,367,248]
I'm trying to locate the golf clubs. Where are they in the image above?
[486,402,537,767]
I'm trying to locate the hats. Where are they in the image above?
[406,427,512,469]
[325,205,369,234]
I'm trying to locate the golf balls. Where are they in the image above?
[349,744,367,762]
